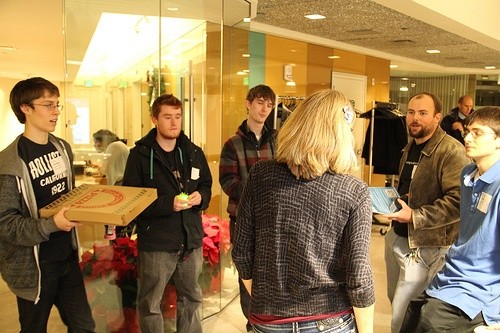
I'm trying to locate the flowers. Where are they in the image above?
[198,213,232,288]
[79,236,138,316]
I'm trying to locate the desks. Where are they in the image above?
[75,171,107,185]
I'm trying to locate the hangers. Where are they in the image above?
[350,100,406,118]
[278,95,306,111]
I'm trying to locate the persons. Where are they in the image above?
[0,77,96,333]
[91,94,213,333]
[218,85,279,332]
[382,92,500,333]
[232,89,376,333]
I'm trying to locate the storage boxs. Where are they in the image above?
[39,183,158,227]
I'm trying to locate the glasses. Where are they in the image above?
[27,103,63,111]
[463,129,498,138]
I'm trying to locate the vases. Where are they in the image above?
[211,269,224,292]
[106,307,139,333]
[160,284,177,319]
